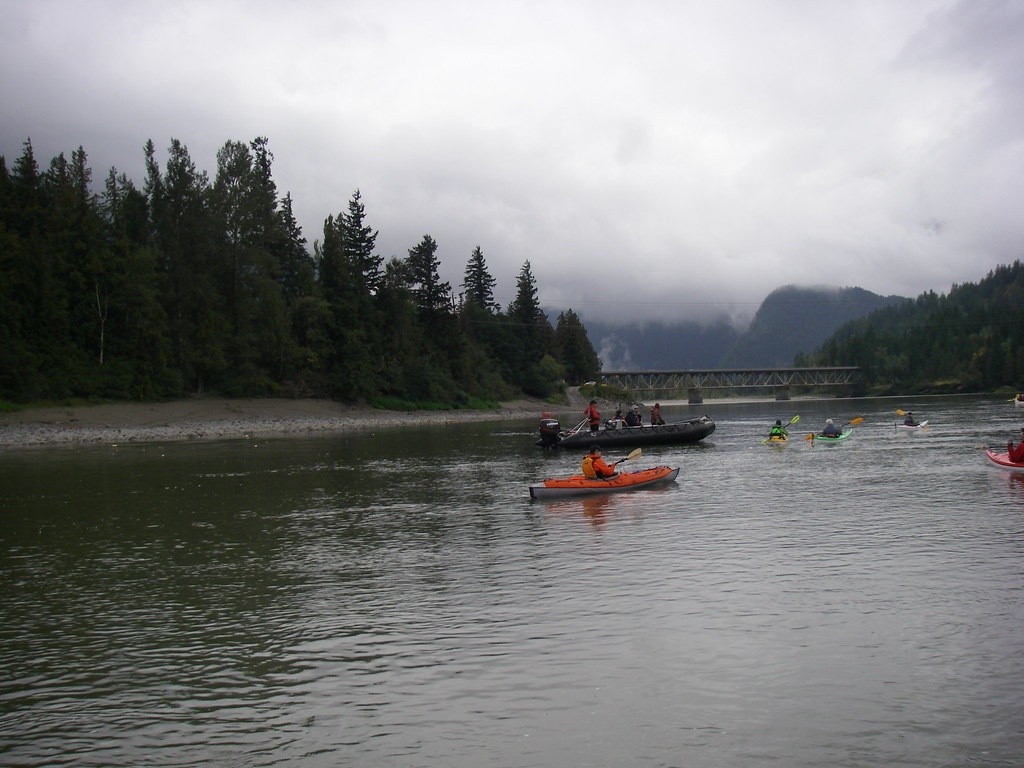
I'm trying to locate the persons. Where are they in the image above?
[625,407,642,426]
[612,410,625,426]
[904,411,919,425]
[581,445,616,479]
[649,403,665,425]
[584,400,601,431]
[1007,427,1024,462]
[823,419,842,438]
[768,420,788,440]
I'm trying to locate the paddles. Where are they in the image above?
[896,408,926,429]
[804,417,864,443]
[609,446,642,468]
[762,413,800,444]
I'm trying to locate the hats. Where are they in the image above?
[590,445,604,451]
[590,400,596,405]
[632,405,638,409]
[908,412,912,414]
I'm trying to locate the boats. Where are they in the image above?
[1015,397,1024,408]
[813,427,854,441]
[984,448,1024,473]
[767,433,788,448]
[893,418,930,430]
[535,414,716,451]
[528,463,680,501]
